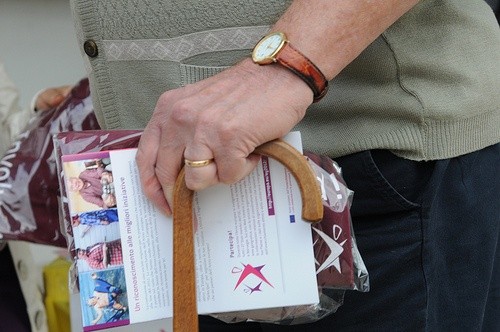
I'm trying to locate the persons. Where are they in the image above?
[71,210,119,227]
[66,167,115,207]
[85,277,127,326]
[62,1,499,332]
[71,239,124,270]
[1,53,72,163]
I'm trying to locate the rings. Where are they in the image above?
[183,158,214,167]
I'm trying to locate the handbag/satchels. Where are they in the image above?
[1,74,101,250]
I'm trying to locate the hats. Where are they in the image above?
[75,248,80,261]
[72,213,79,225]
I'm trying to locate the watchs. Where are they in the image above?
[250,33,334,101]
